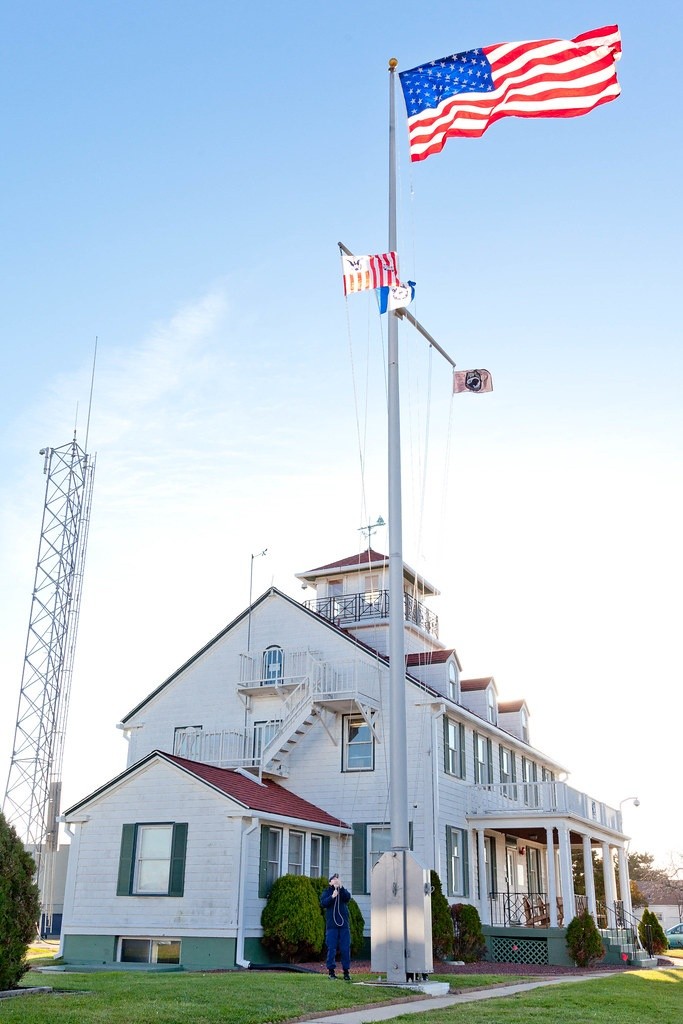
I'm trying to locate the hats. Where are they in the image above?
[329,873,339,882]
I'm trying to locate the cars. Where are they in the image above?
[666,924,683,952]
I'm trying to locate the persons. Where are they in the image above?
[320,873,351,980]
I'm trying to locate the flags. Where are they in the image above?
[379,280,416,314]
[342,252,400,295]
[453,369,494,394]
[399,25,623,162]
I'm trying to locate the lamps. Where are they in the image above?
[519,847,525,855]
[529,834,538,840]
[620,796,640,810]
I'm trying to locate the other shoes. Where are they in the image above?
[343,971,351,980]
[329,970,337,980]
